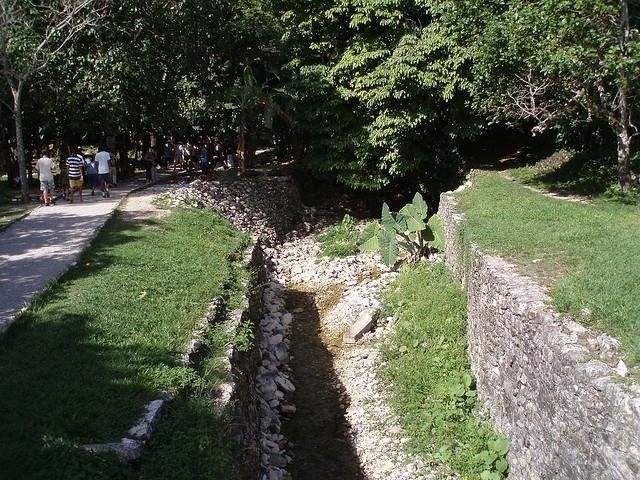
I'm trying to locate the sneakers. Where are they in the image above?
[107,191,111,198]
[45,203,49,206]
[102,192,106,198]
[49,200,56,204]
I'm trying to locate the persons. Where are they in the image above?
[35,138,234,206]
